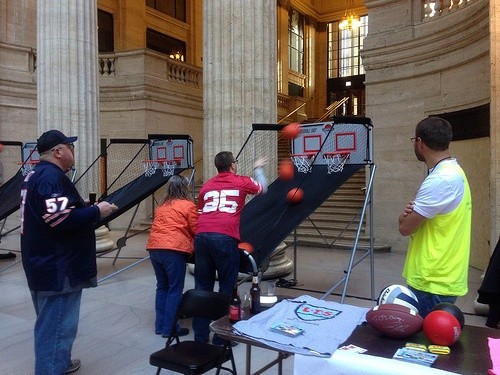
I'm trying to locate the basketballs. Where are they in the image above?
[278,160,293,180]
[286,187,303,203]
[282,123,301,141]
[238,241,253,254]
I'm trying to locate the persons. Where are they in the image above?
[194,151,269,347]
[21,130,117,374]
[398,117,472,308]
[146,176,199,337]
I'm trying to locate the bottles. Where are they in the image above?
[240,292,250,320]
[249,272,261,314]
[228,285,241,324]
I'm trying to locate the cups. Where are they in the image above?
[267,279,276,296]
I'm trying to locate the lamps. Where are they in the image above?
[340,0,360,29]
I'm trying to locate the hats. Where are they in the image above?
[37,130,78,153]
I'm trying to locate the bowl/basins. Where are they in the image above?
[260,297,278,307]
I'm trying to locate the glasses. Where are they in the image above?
[175,173,184,181]
[68,142,74,147]
[410,137,417,143]
[232,160,239,164]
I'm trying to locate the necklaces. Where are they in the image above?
[431,156,452,173]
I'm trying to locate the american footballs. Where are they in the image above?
[365,303,423,336]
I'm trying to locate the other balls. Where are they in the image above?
[431,302,464,332]
[377,284,419,313]
[423,310,460,347]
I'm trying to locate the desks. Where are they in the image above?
[208,295,500,375]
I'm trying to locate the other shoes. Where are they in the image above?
[155,332,162,334]
[65,358,81,373]
[163,327,189,337]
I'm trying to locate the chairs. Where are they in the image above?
[150,288,236,375]
